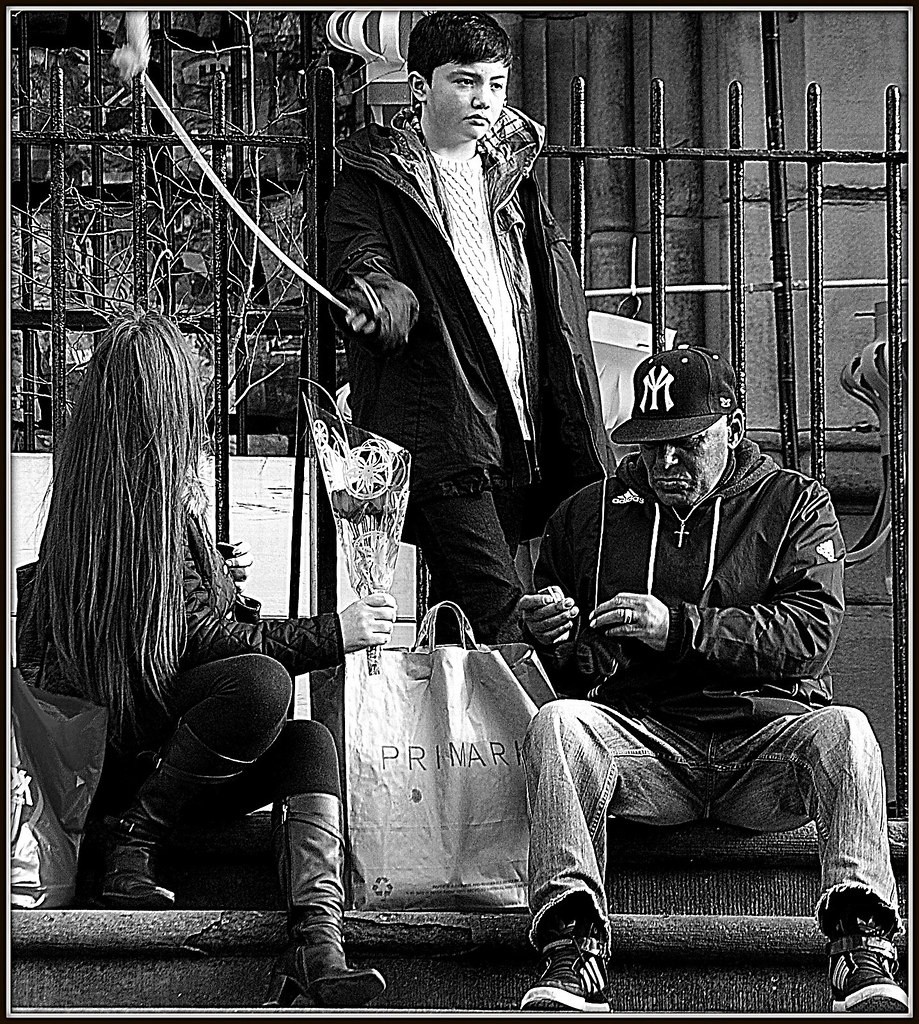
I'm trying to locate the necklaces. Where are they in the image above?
[669,486,719,548]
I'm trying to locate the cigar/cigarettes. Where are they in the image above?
[545,586,559,605]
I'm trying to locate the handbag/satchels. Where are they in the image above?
[341,599,558,915]
[7,661,108,909]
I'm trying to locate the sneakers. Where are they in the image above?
[825,924,908,1012]
[515,917,614,1017]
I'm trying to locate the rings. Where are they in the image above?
[623,606,633,626]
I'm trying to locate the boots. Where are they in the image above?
[97,717,255,909]
[265,792,388,1008]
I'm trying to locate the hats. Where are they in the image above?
[610,346,736,444]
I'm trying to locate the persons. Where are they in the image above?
[509,346,909,1017]
[14,310,398,1008]
[324,10,615,646]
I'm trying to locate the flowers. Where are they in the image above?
[298,374,412,676]
